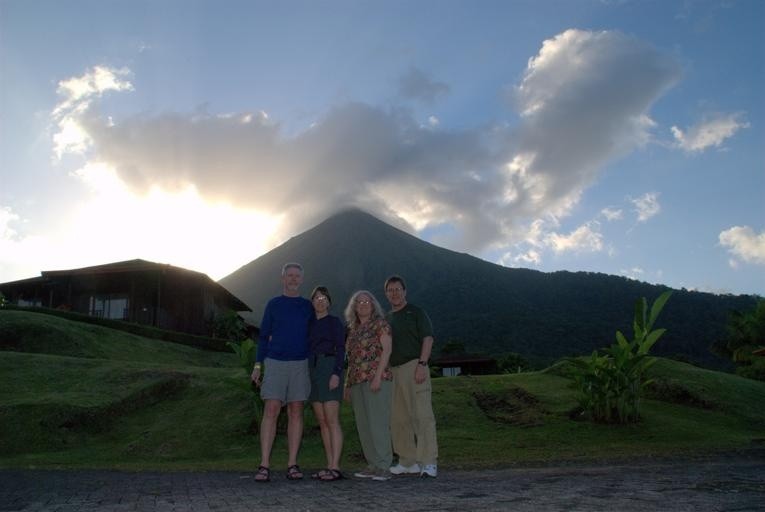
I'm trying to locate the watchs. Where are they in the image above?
[418,361,427,366]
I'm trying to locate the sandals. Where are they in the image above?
[287,464,304,480]
[254,466,270,482]
[312,468,342,482]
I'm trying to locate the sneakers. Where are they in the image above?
[353,471,392,481]
[421,464,438,478]
[390,464,420,475]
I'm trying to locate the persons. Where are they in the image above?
[344,291,394,482]
[251,262,316,481]
[308,284,350,478]
[384,275,440,478]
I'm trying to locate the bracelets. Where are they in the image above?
[254,364,260,369]
[345,383,352,389]
